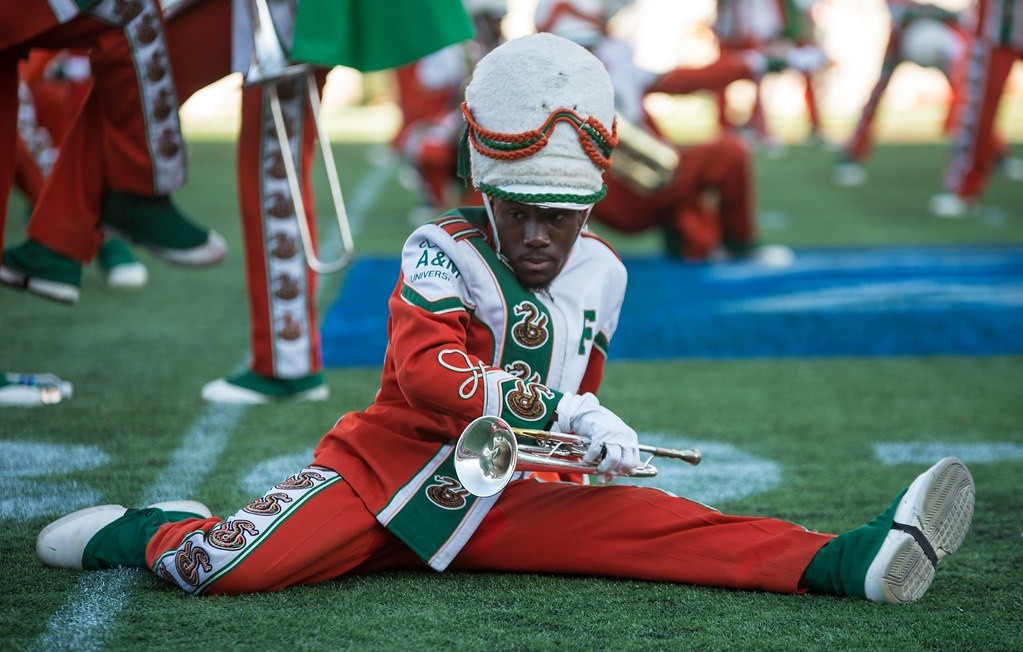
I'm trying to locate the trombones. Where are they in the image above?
[242,0,355,276]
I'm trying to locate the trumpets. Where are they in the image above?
[453,413,702,499]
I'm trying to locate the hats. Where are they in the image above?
[456,30,618,208]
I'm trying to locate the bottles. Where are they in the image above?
[0,369,75,408]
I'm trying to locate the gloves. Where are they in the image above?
[556,392,643,473]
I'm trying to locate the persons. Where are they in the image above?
[705,0,832,155]
[0,0,470,408]
[928,1,1023,222]
[822,0,1023,197]
[36,89,979,610]
[524,0,794,272]
[10,25,147,289]
[389,1,537,229]
[0,1,234,299]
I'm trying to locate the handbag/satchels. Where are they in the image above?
[925,192,978,219]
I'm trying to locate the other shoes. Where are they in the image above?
[150,228,226,266]
[36,500,213,570]
[865,456,975,602]
[832,156,866,189]
[198,377,329,404]
[99,259,151,290]
[0,261,80,307]
[749,243,791,266]
